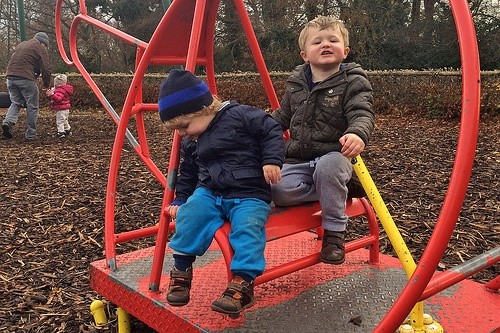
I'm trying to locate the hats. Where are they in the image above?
[34,32,49,48]
[158,68,214,123]
[54,74,67,85]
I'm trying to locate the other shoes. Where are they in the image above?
[65,131,72,137]
[1,123,13,139]
[26,135,37,140]
[54,132,65,141]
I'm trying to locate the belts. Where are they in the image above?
[7,77,25,80]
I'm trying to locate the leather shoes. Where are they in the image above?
[345,178,367,199]
[320,230,346,264]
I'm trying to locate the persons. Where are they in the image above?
[159,69,286,314]
[2,32,52,140]
[267,16,375,265]
[46,74,74,137]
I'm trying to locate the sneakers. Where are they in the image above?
[211,275,255,314]
[167,266,193,307]
